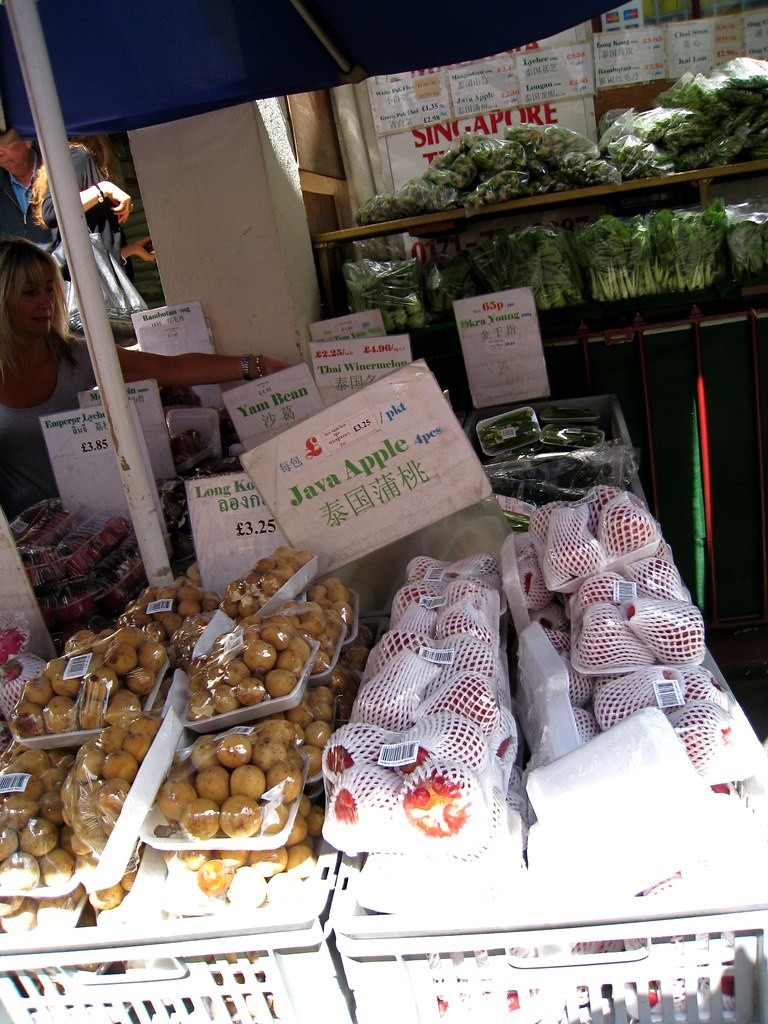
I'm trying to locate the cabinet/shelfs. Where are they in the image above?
[318,154,768,674]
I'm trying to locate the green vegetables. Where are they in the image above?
[343,57,768,332]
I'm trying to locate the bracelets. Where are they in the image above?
[94,184,105,202]
[256,355,263,377]
[121,255,127,265]
[242,354,250,381]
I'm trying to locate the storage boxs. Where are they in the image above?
[0,393,768,1023]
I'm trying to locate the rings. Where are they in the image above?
[130,203,133,212]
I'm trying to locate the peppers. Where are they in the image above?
[481,407,602,451]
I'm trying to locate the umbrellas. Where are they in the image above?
[0,0,629,589]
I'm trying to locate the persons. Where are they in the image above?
[0,130,58,252]
[1,240,290,522]
[32,134,155,282]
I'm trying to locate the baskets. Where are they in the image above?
[330,849,768,1024]
[0,840,353,1024]
